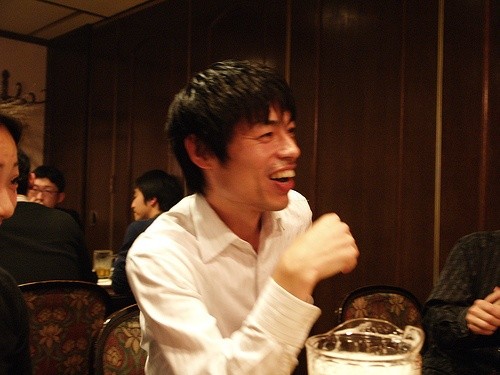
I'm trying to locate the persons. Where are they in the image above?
[126,56,361,374]
[0,147,100,290]
[0,101,34,375]
[106,168,185,316]
[421,228,500,374]
[29,164,85,231]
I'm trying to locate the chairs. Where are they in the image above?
[18,279,423,375]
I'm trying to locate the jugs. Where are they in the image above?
[304,318,425,375]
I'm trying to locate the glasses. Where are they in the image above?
[29,185,60,196]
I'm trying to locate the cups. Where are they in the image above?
[93,250,117,278]
